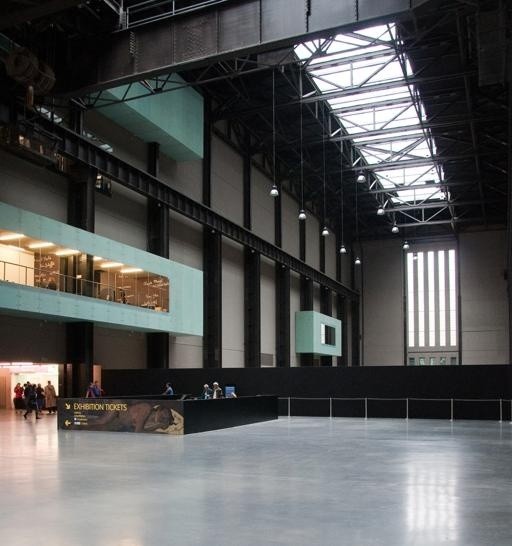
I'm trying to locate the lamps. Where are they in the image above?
[270,62,410,265]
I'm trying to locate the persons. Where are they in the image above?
[195,384,213,400]
[163,382,173,395]
[208,382,222,399]
[85,380,102,398]
[14,380,56,420]
